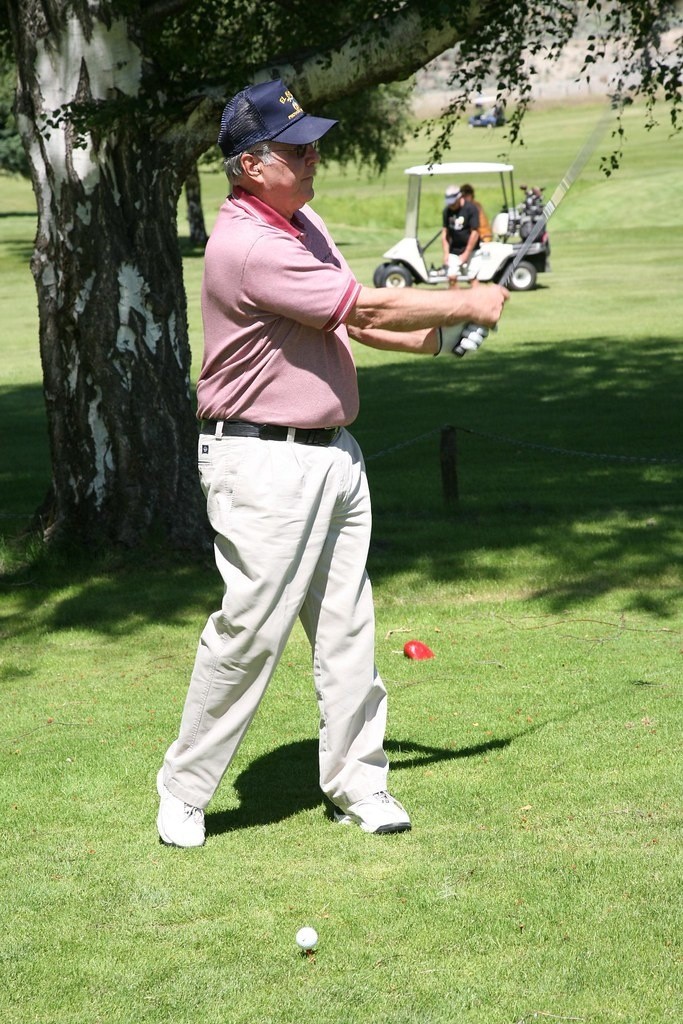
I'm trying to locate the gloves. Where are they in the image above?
[434,321,488,358]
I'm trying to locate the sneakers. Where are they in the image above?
[156,766,206,848]
[334,791,411,834]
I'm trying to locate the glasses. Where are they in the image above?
[244,140,317,159]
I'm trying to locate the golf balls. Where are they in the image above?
[296,927,318,949]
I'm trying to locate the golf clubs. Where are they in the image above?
[453,110,613,355]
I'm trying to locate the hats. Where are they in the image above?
[218,80,339,159]
[445,186,463,205]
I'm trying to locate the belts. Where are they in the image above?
[199,419,336,447]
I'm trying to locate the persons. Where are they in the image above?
[442,186,482,290]
[460,183,493,243]
[155,85,508,849]
